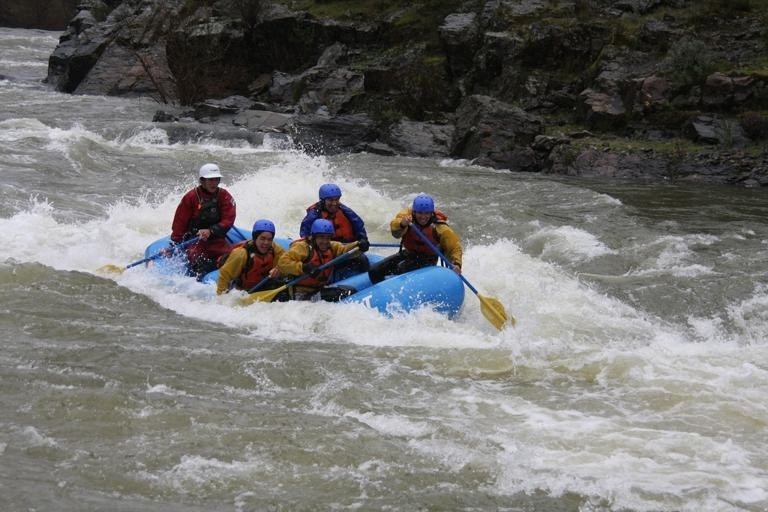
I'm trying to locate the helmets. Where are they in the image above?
[413,195,434,213]
[252,219,276,241]
[319,184,341,199]
[310,219,335,235]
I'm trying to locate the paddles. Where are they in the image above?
[95,237,199,279]
[408,222,517,333]
[233,248,362,311]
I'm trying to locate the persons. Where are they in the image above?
[215,220,288,296]
[277,220,370,303]
[369,194,461,286]
[159,162,236,283]
[300,185,369,274]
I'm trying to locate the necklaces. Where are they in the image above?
[194,186,204,211]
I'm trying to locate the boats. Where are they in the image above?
[147,226,464,323]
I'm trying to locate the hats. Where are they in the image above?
[199,163,223,179]
